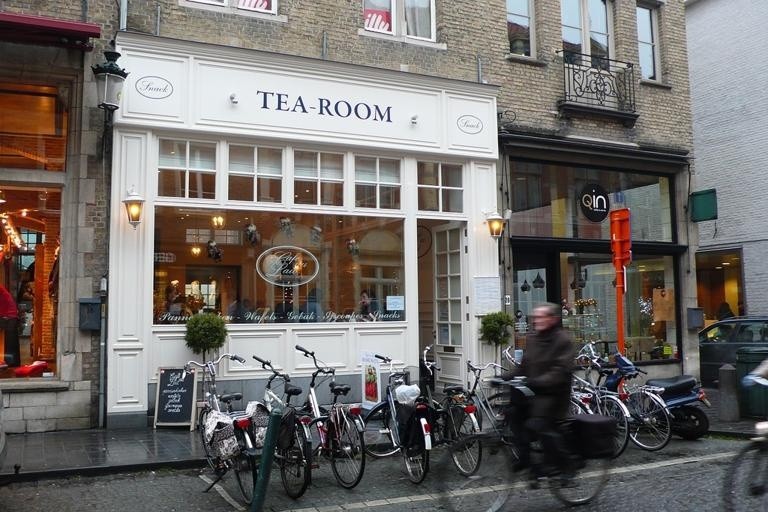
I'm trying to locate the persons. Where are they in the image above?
[163,285,185,324]
[716,302,736,335]
[299,288,323,321]
[358,289,379,322]
[741,356,768,388]
[491,303,574,474]
[738,301,744,316]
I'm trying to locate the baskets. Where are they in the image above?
[403,366,426,396]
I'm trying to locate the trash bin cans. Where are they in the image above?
[735,346,768,419]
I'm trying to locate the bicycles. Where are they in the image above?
[572,342,665,447]
[458,353,535,464]
[186,353,257,507]
[247,354,316,501]
[366,354,437,482]
[438,413,610,505]
[496,344,627,445]
[294,345,372,486]
[420,345,488,477]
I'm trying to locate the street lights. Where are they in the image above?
[83,40,129,428]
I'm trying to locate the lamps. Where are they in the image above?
[520,280,531,293]
[570,268,587,289]
[532,271,546,288]
[119,186,146,230]
[90,39,129,152]
[482,211,509,238]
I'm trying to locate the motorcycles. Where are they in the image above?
[598,353,713,435]
[722,353,767,507]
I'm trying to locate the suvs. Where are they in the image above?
[696,315,768,388]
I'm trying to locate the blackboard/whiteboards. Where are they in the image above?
[157,369,194,423]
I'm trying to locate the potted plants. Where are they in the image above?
[183,311,228,426]
[478,312,515,407]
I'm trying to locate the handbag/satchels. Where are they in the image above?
[277,406,295,450]
[246,401,271,448]
[396,406,425,457]
[204,410,241,461]
